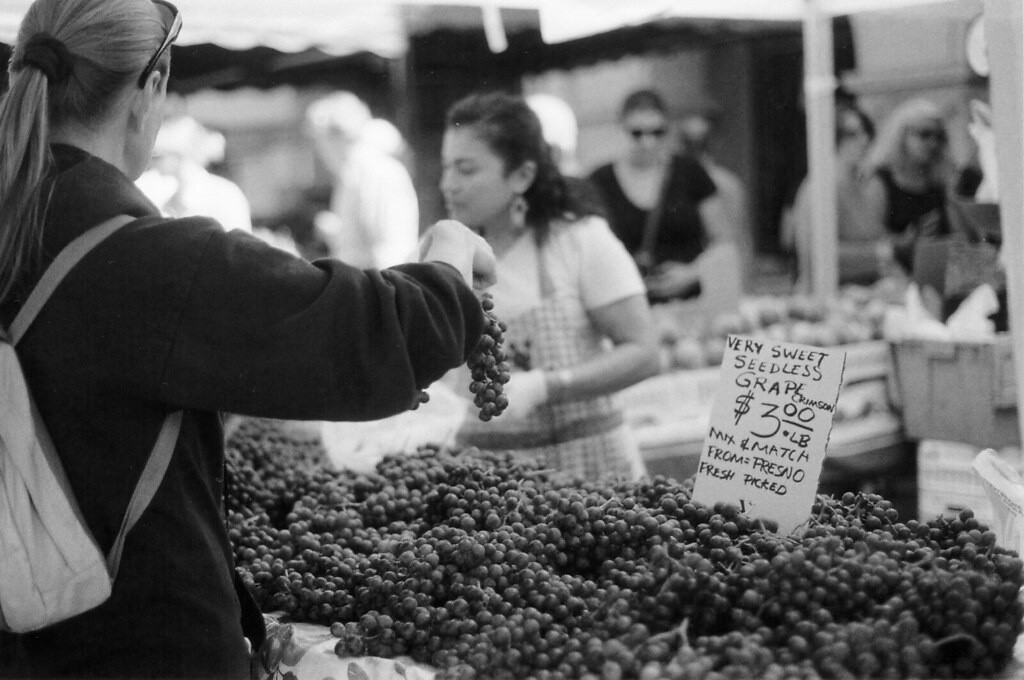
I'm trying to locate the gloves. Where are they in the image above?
[463,367,547,422]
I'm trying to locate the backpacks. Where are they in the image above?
[0,215,181,633]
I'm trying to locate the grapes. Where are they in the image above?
[222,293,1024,680]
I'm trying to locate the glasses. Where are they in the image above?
[137,0,182,90]
[909,130,948,144]
[627,125,670,139]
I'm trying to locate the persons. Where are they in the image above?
[0,1,499,680]
[439,92,661,483]
[302,90,422,269]
[794,108,886,286]
[864,98,967,279]
[669,94,746,298]
[590,90,733,306]
[150,118,253,234]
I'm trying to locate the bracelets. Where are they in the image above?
[558,369,572,404]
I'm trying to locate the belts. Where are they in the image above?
[457,411,623,450]
